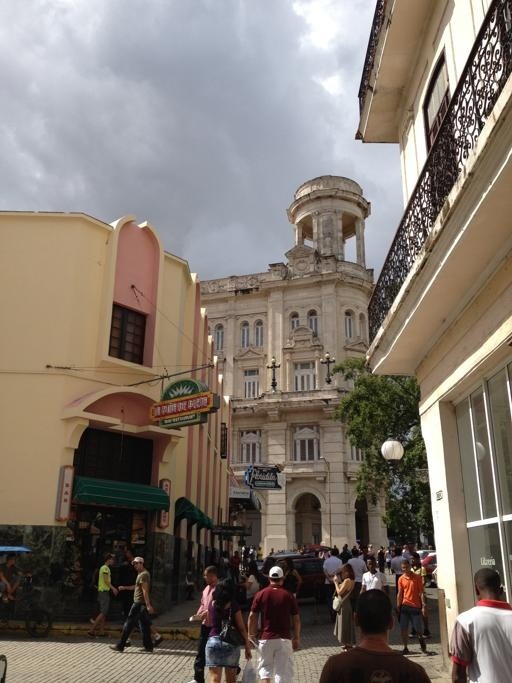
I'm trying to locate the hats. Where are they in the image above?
[131,557,144,565]
[268,566,283,578]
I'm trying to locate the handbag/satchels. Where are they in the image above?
[332,596,342,611]
[219,622,243,647]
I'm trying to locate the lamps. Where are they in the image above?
[381,430,428,484]
[320,352,335,384]
[266,356,280,389]
[476,441,486,463]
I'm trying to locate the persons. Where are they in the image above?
[315,541,438,683]
[84,544,164,654]
[0,553,25,604]
[449,567,512,683]
[183,543,309,683]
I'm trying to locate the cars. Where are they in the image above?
[257,550,326,603]
[416,549,437,585]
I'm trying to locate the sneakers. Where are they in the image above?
[87,630,162,652]
